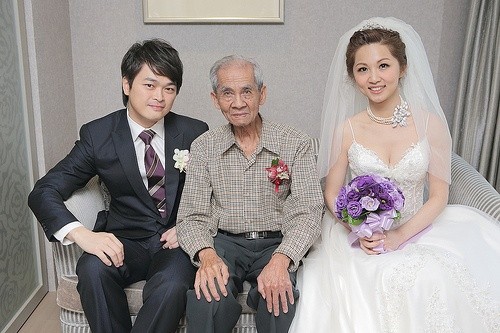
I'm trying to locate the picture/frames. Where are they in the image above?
[142,0,285,25]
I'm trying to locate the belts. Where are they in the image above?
[217,228,285,240]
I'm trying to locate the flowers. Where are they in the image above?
[336,172,406,255]
[173,148,190,174]
[264,157,290,193]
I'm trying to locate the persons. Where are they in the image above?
[176,54,325,333]
[28,38,210,333]
[289,16,500,333]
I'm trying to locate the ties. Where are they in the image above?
[138,129,167,219]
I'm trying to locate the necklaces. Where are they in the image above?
[366,94,411,128]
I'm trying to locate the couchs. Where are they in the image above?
[51,152,500,333]
